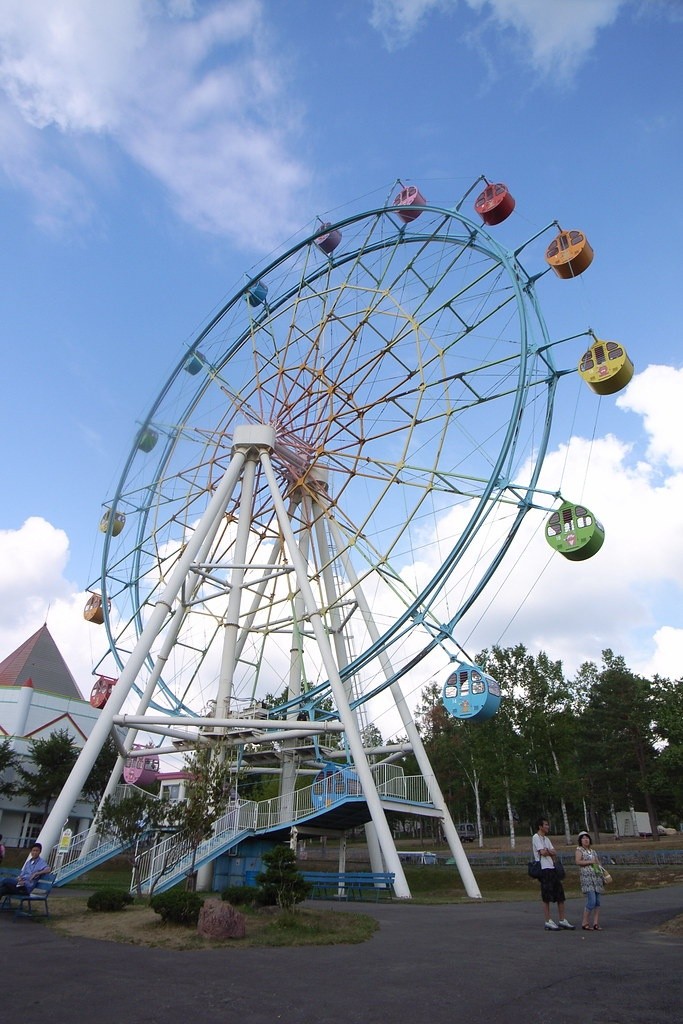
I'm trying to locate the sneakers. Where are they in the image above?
[558,919,576,930]
[544,919,561,931]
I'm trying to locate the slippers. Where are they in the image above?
[582,924,593,930]
[594,924,602,930]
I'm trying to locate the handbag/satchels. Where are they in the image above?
[598,865,612,885]
[528,861,541,879]
[554,862,565,879]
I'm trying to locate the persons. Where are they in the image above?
[575,831,604,932]
[0,833,52,901]
[532,818,575,931]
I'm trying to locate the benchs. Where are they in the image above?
[300,872,396,903]
[0,867,58,923]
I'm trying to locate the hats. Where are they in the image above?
[578,831,589,836]
